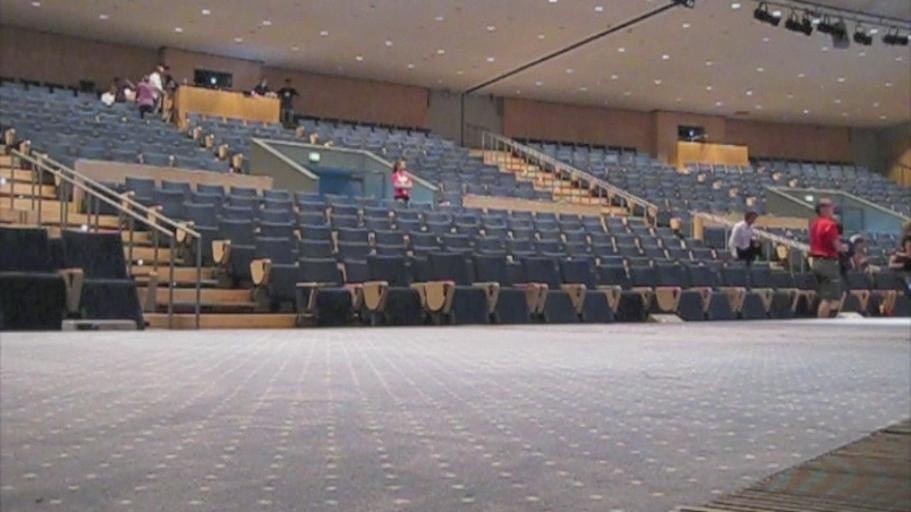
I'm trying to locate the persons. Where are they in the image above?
[275,78,303,124]
[111,63,190,119]
[391,157,414,199]
[254,75,274,98]
[728,210,761,263]
[808,198,911,317]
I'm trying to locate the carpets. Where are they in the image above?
[0,317,911,510]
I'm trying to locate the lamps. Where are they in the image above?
[750,2,909,54]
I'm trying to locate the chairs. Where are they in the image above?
[1,78,911,329]
